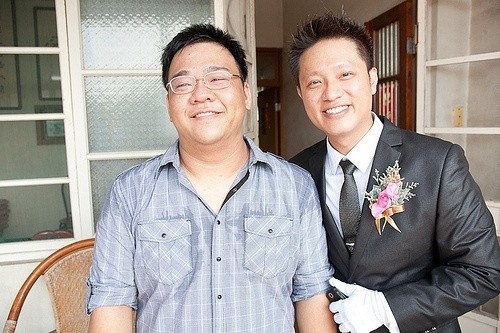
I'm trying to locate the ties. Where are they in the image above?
[338,159,361,258]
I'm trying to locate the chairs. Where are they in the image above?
[3,237,136,333]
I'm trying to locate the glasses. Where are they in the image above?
[165,71,243,95]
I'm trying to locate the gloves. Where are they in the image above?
[326,277,387,333]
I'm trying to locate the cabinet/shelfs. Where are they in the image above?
[417,0,500,333]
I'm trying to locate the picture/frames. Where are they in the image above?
[35,104,65,145]
[0,0,22,111]
[33,6,63,101]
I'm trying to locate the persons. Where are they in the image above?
[281,16,500,333]
[83,22,338,332]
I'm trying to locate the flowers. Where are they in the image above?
[363,161,418,234]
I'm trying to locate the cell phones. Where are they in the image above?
[326,288,346,304]
